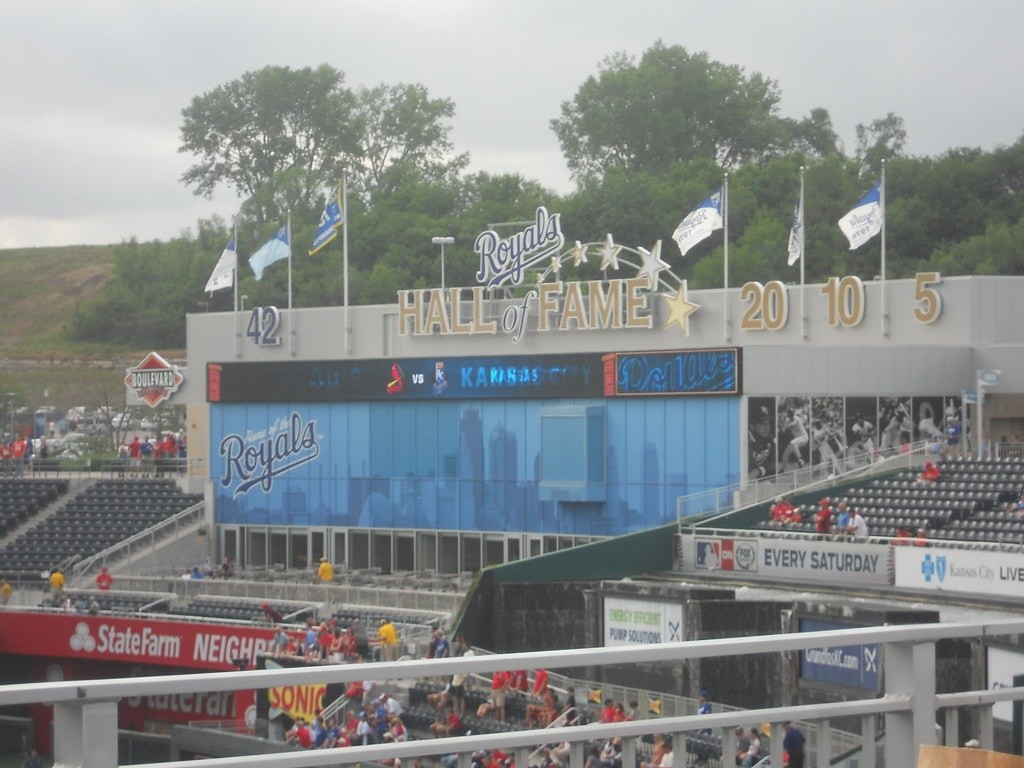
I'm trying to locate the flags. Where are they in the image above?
[838,178,881,251]
[787,183,801,266]
[248,225,290,280]
[672,186,724,256]
[308,180,345,255]
[205,235,238,292]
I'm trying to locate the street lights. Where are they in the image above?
[8,393,19,444]
[433,236,455,291]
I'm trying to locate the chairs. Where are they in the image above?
[38,589,722,761]
[0,477,205,584]
[738,455,1024,556]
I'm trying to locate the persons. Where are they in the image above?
[44,417,77,440]
[319,557,332,586]
[770,497,927,547]
[181,555,235,579]
[813,409,844,478]
[778,407,808,475]
[117,429,187,479]
[878,407,906,460]
[0,567,112,616]
[846,412,876,466]
[272,618,805,768]
[0,434,49,473]
[746,402,775,481]
[921,417,1024,517]
[21,749,43,768]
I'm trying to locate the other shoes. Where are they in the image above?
[778,469,784,473]
[798,458,804,467]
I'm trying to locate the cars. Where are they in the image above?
[0,401,182,459]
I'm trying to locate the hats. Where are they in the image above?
[320,557,327,561]
[774,497,783,503]
[750,404,773,423]
[358,711,367,718]
[924,462,932,467]
[434,628,442,636]
[820,500,828,504]
[700,691,708,698]
[379,693,388,700]
[370,698,380,704]
[856,412,862,419]
